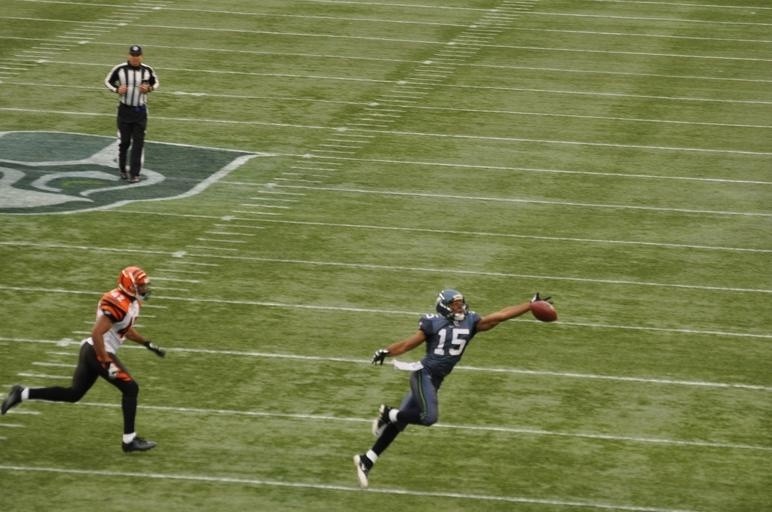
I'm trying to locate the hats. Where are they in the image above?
[128,44,143,57]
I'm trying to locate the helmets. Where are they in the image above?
[434,289,470,323]
[116,265,152,301]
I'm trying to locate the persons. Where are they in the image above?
[353,290,554,487]
[103,45,159,182]
[1,266,166,452]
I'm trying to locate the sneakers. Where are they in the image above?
[371,404,394,437]
[352,454,374,489]
[121,436,156,453]
[0,384,25,415]
[120,172,140,183]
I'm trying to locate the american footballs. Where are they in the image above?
[530,301,558,322]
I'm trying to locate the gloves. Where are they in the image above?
[529,292,554,305]
[104,360,122,379]
[143,340,166,358]
[371,349,390,366]
